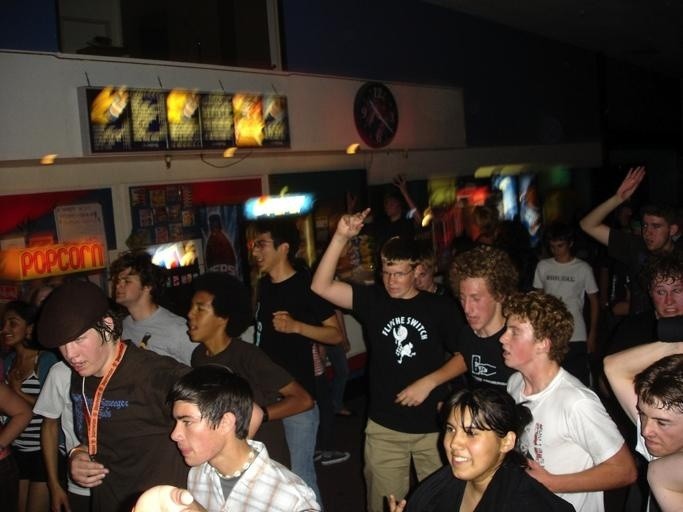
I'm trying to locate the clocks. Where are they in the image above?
[354,80,398,149]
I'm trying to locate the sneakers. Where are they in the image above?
[313,449,322,462]
[321,451,351,465]
[331,407,357,417]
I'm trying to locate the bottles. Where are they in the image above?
[205,215,238,275]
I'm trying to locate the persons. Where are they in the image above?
[3,165,683,512]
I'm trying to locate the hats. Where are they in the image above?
[35,280,109,348]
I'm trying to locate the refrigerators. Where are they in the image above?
[192,192,318,314]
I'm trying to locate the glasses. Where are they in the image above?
[380,267,415,277]
